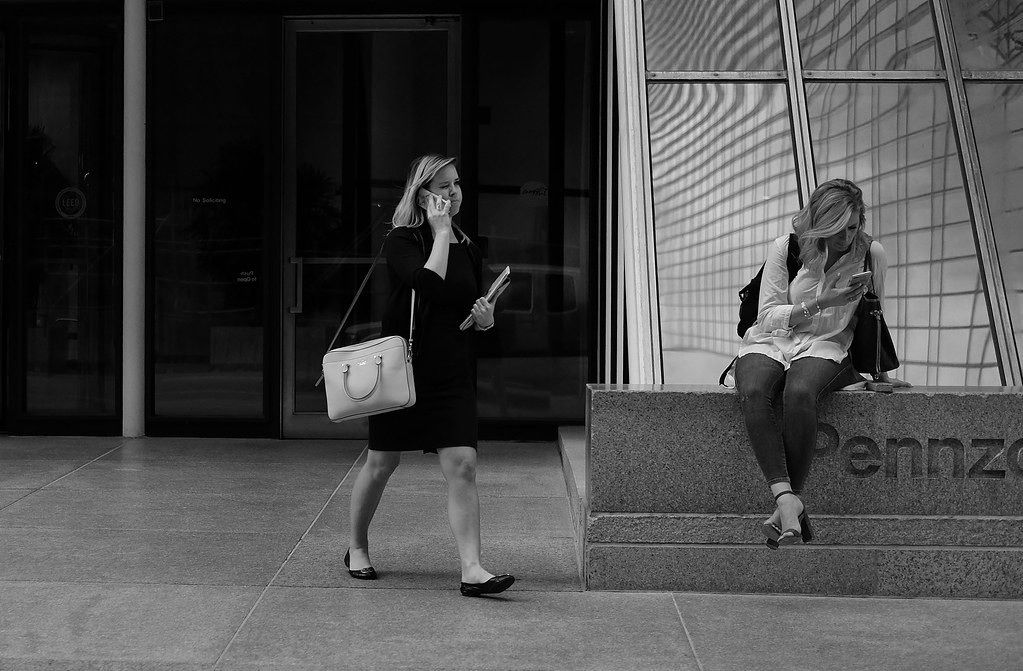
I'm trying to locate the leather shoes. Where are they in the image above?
[344,547,376,580]
[460,574,516,597]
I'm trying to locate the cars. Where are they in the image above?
[347,265,591,396]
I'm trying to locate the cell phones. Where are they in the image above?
[849,271,873,294]
[416,189,452,211]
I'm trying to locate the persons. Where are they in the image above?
[344,154,515,595]
[732,177,912,550]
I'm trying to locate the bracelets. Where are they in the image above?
[801,302,813,321]
[816,296,822,313]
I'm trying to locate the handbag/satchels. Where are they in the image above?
[735,232,801,340]
[315,335,417,423]
[851,250,899,374]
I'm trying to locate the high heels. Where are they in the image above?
[775,491,815,543]
[761,506,782,551]
